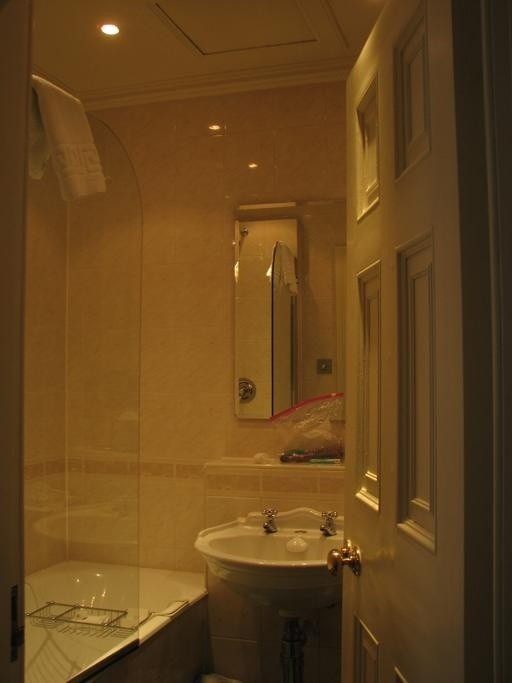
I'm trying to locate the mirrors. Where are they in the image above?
[234,218,298,419]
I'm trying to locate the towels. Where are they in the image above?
[266,241,297,297]
[31,75,107,201]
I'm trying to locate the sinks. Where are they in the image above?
[196,518,352,619]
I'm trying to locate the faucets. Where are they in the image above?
[263,505,280,533]
[319,510,341,537]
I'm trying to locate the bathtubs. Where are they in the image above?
[23,560,204,682]
